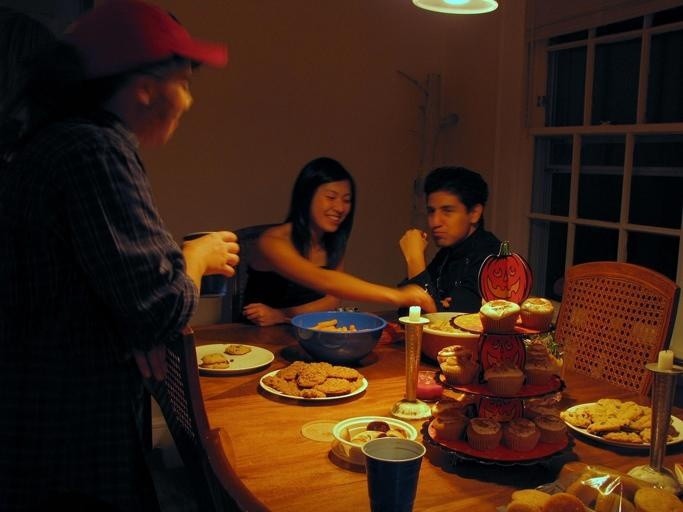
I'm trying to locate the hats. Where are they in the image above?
[65,1,229,78]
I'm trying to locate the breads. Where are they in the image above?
[507,461,683,512]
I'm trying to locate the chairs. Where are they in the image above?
[553,261,680,395]
[219,225,278,323]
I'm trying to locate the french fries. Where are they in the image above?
[309,318,361,333]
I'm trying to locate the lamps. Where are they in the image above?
[412,0,498,15]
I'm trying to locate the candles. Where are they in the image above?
[658,349,674,369]
[409,305,421,321]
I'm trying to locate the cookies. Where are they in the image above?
[560,398,678,443]
[201,345,228,368]
[262,360,359,398]
[224,342,249,354]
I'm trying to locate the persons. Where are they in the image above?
[0,0,241,512]
[398,166,504,330]
[239,157,437,328]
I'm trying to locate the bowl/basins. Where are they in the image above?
[289,312,387,362]
[331,415,416,456]
[416,312,482,365]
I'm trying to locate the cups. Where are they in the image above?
[182,230,228,299]
[362,439,427,510]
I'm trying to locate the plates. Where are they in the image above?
[190,343,275,376]
[564,402,683,449]
[257,367,369,402]
[326,442,365,468]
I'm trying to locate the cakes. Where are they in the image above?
[479,298,520,333]
[520,296,554,330]
[430,339,566,451]
